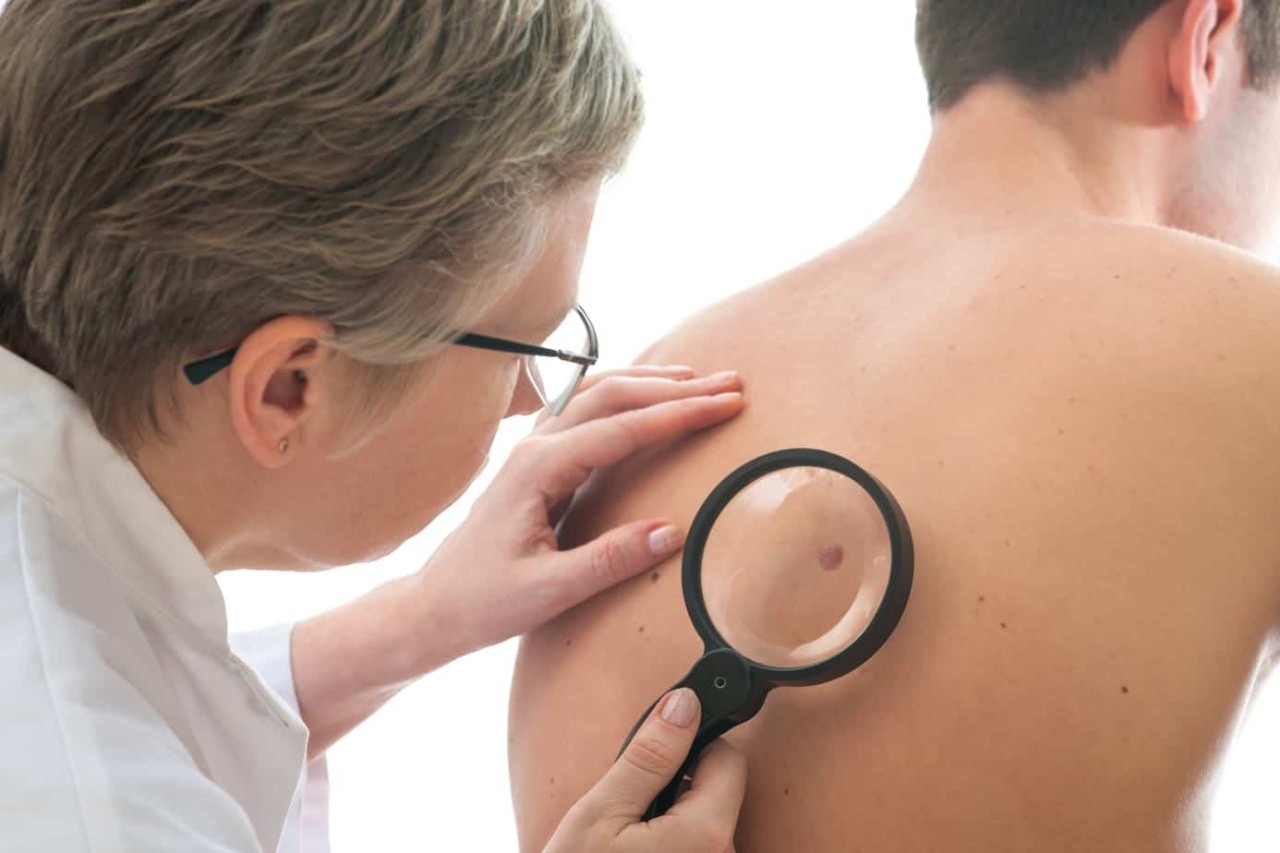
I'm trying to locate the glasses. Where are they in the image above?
[183,304,598,419]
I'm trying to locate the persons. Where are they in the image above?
[0,0,749,852]
[506,0,1280,853]
